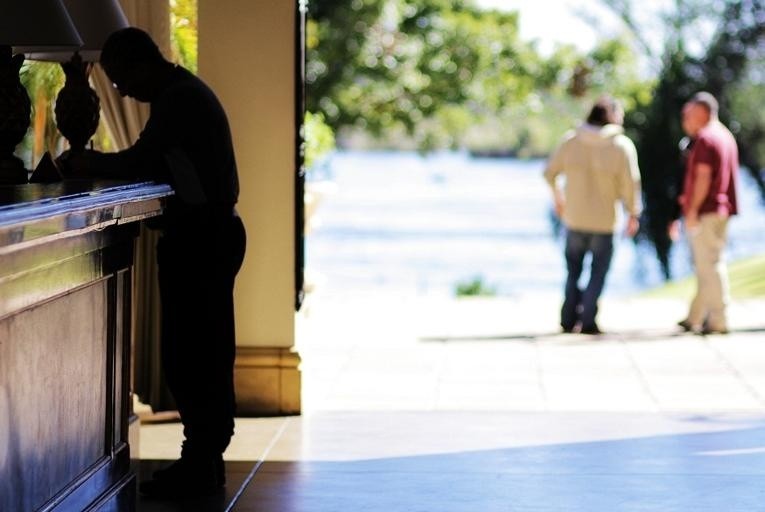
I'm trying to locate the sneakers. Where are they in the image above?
[137,458,227,497]
[558,318,734,338]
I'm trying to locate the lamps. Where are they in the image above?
[2,1,136,184]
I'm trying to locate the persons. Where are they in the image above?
[56,23,246,489]
[543,92,645,337]
[665,91,741,336]
